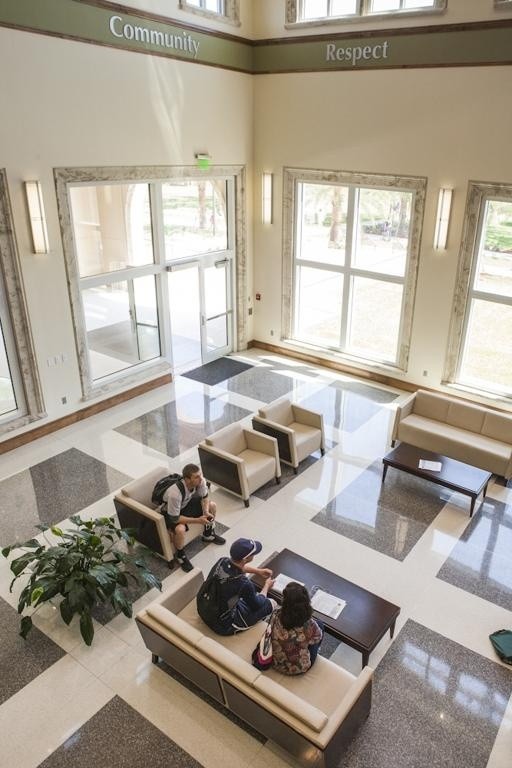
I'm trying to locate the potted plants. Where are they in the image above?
[1,514,163,647]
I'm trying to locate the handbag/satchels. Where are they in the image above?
[250,608,279,670]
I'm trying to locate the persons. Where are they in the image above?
[196,537,277,636]
[267,582,323,677]
[156,462,226,574]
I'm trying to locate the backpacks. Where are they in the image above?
[151,472,186,504]
[197,572,229,633]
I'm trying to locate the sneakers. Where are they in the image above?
[174,552,194,574]
[199,532,227,546]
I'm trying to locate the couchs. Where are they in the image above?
[251,398,325,474]
[113,467,216,570]
[390,389,512,487]
[197,422,282,508]
[135,566,373,768]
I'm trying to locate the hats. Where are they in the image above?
[229,537,263,563]
